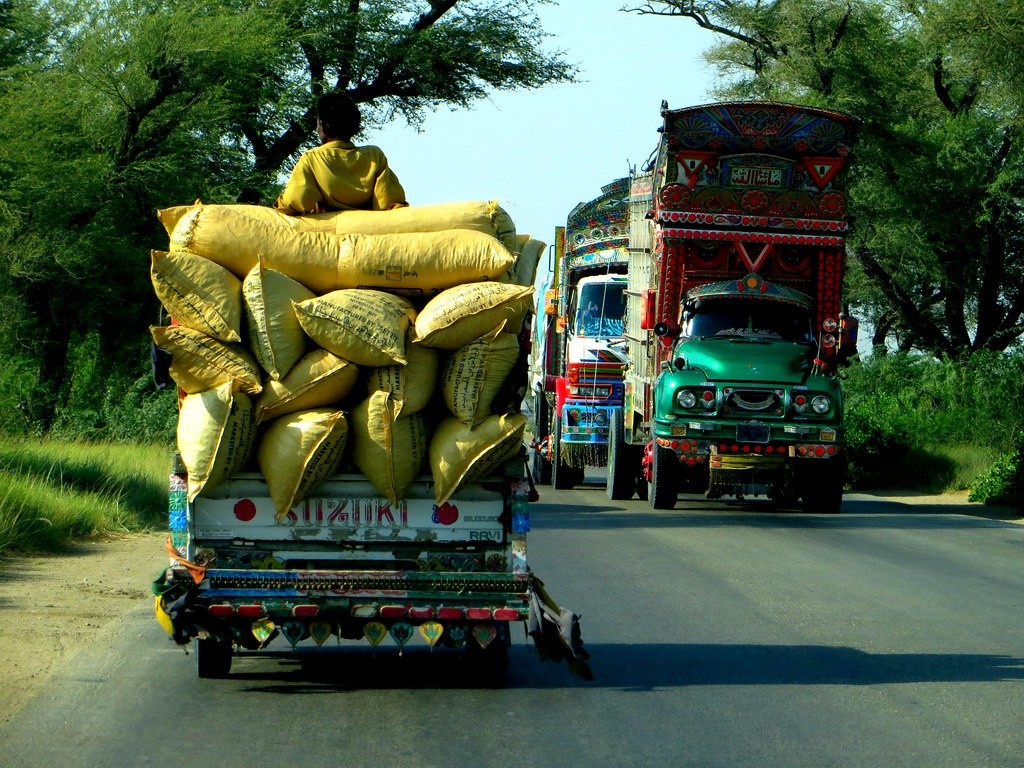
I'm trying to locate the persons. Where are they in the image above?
[580,294,607,320]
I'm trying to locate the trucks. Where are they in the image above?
[185,478,533,682]
[528,97,868,516]
